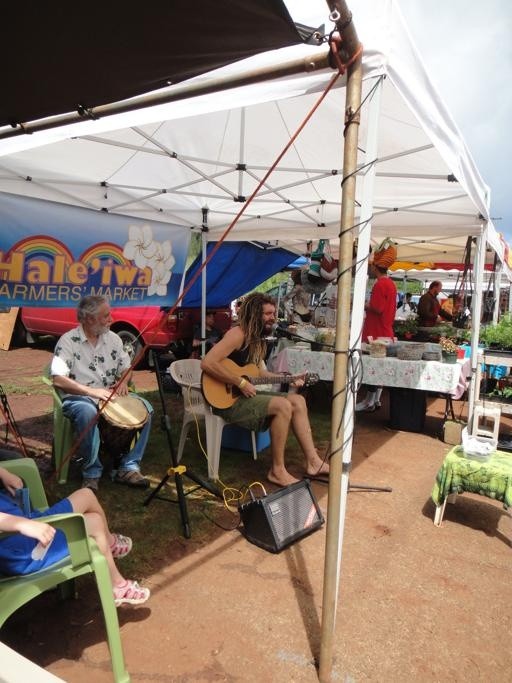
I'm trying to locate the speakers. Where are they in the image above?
[237,477,327,555]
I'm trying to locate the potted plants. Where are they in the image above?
[439,337,466,365]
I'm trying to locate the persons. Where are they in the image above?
[49,292,155,493]
[192,310,224,357]
[1,465,151,610]
[201,291,330,490]
[287,246,462,412]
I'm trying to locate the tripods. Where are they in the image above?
[139,354,227,540]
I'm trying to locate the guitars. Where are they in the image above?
[201,357,319,415]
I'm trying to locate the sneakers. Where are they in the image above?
[111,534,133,558]
[115,471,150,488]
[113,579,150,607]
[81,479,99,493]
[356,398,382,413]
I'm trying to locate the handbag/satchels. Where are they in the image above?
[295,311,313,322]
[301,248,337,293]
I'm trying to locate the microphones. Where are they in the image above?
[152,335,192,356]
[271,323,302,343]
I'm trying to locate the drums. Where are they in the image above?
[99,393,150,461]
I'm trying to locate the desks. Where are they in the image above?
[277,344,469,442]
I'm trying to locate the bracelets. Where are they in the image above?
[237,376,248,388]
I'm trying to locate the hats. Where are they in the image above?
[291,269,301,279]
[373,246,396,269]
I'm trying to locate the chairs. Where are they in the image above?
[1,459,129,680]
[169,357,258,481]
[43,363,135,484]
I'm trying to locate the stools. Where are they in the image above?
[432,444,511,528]
